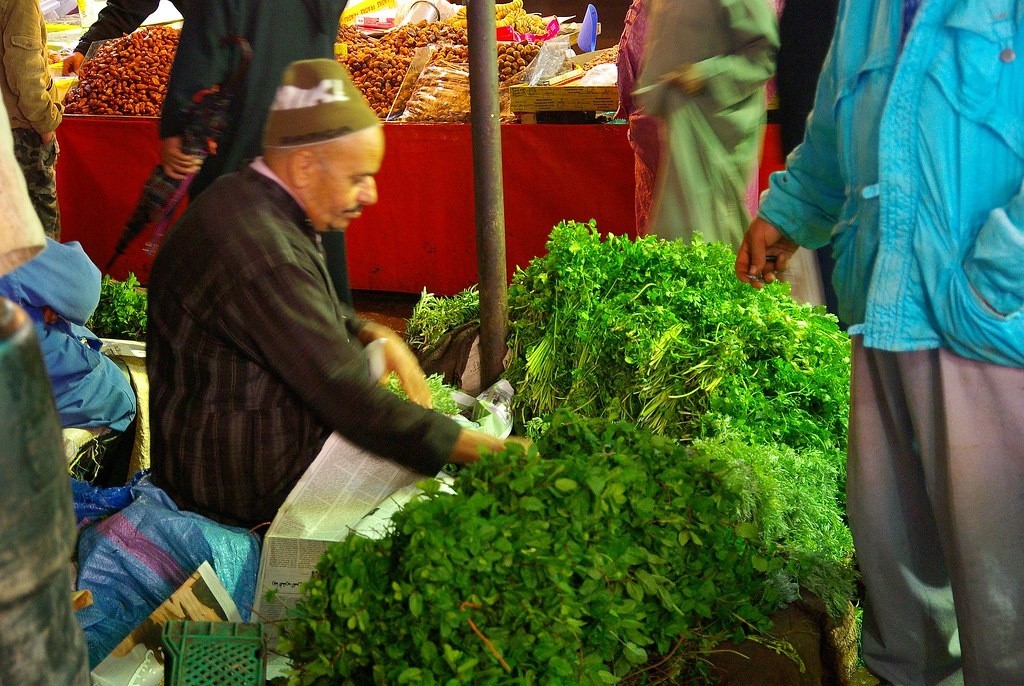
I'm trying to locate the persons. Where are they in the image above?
[613,0,784,242]
[735,0,1024,680]
[631,0,779,253]
[147,60,506,534]
[0,0,355,306]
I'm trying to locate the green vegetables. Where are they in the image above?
[82,272,150,341]
[218,214,861,686]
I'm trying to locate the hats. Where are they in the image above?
[262,59,381,149]
